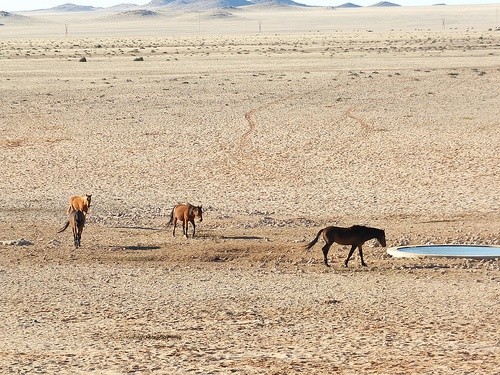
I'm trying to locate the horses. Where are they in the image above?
[299,226,388,267]
[165,204,203,238]
[57,193,93,249]
